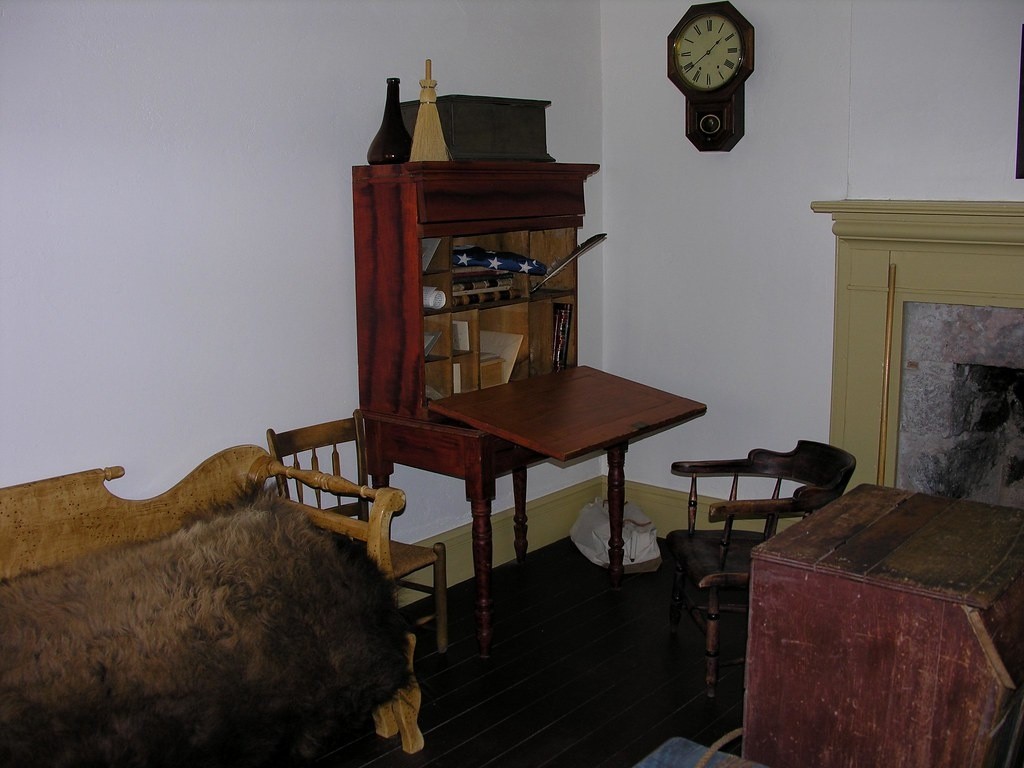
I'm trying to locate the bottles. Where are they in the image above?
[366,77,413,166]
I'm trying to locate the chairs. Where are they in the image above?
[667,440,857,697]
[265,409,446,655]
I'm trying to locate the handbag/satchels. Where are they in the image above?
[569,498,663,574]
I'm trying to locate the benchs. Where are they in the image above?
[1,444,425,768]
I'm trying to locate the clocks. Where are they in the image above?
[666,0,755,153]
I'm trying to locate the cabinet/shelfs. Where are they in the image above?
[351,161,707,659]
[741,484,1024,768]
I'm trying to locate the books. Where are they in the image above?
[553,303,572,369]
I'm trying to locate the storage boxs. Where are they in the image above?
[478,351,506,389]
[399,93,555,163]
